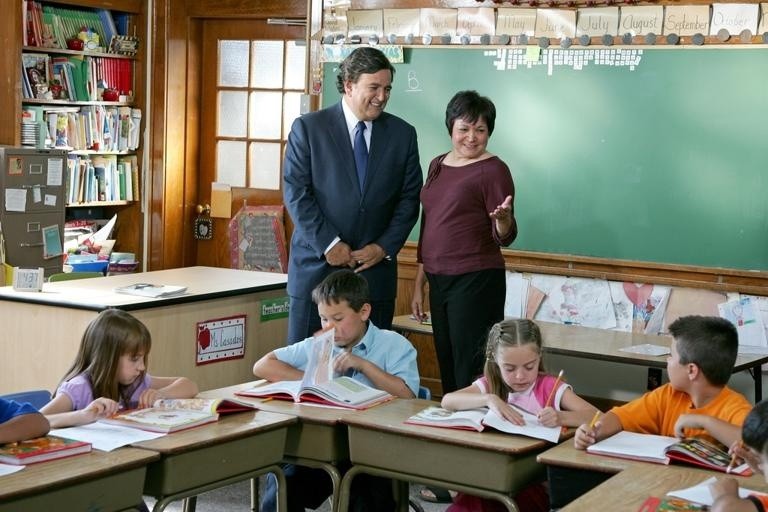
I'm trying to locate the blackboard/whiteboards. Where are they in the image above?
[310,34,768,296]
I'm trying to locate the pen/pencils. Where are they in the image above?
[537,369,563,425]
[726,440,743,473]
[586,411,601,436]
[85,406,123,411]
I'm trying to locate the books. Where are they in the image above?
[0,433,91,465]
[638,496,709,512]
[21,0,140,205]
[118,281,188,297]
[405,404,562,445]
[97,395,260,433]
[234,328,396,411]
[587,428,755,477]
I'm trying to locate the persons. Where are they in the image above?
[282,47,423,346]
[253,269,420,511]
[441,318,603,511]
[707,397,768,512]
[572,315,755,452]
[412,89,518,502]
[35,306,197,512]
[1,397,50,445]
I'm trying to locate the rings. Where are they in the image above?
[357,261,360,264]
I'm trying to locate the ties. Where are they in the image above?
[353,120,370,194]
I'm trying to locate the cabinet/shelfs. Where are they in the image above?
[0,144,74,277]
[0,0,153,272]
